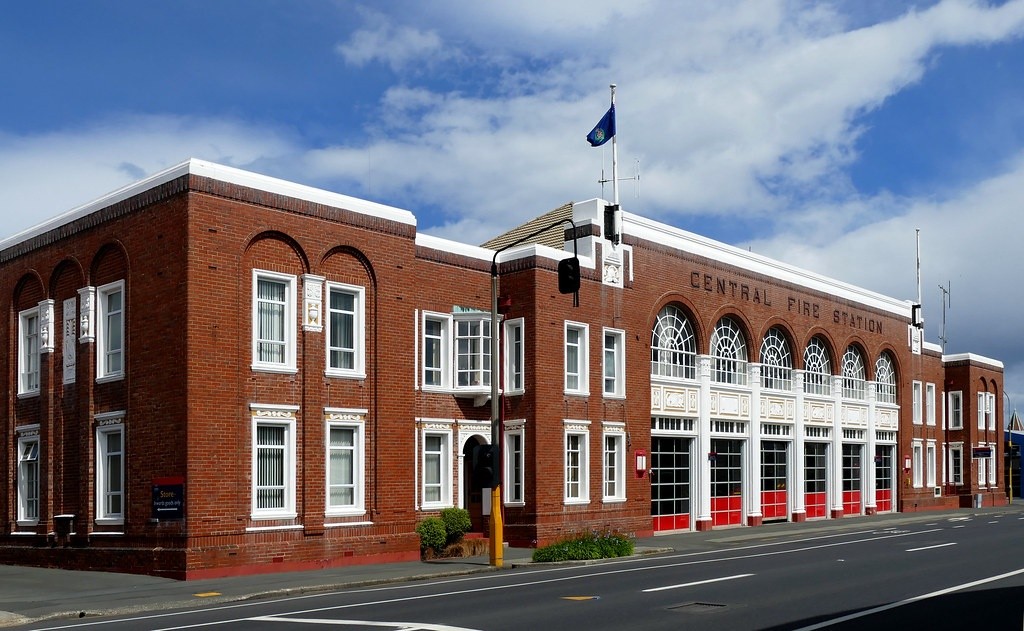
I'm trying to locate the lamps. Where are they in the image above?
[904,455,912,470]
[635,449,647,472]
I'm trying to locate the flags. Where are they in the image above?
[587,103,616,147]
